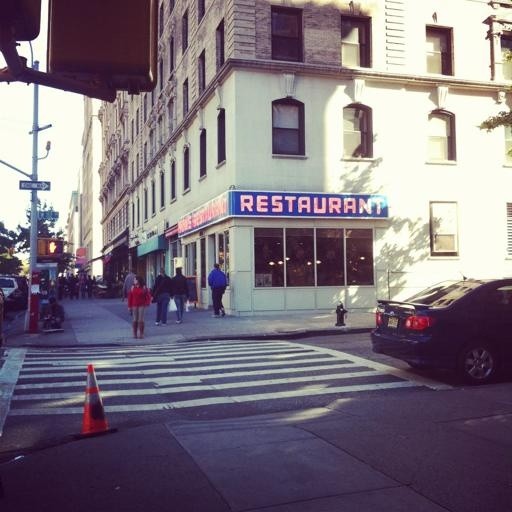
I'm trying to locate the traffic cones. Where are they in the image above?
[79,364,118,440]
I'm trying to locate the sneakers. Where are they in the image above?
[211,307,225,318]
[155,321,168,327]
[175,319,183,323]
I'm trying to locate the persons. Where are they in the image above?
[171,267,188,324]
[127,274,151,339]
[152,268,173,325]
[55,270,135,317]
[206,263,227,318]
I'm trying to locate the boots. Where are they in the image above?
[132,320,144,338]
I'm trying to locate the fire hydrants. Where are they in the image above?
[335,301,348,328]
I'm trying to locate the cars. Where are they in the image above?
[1,274,28,311]
[369,277,511,386]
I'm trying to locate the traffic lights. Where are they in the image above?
[46,238,63,257]
